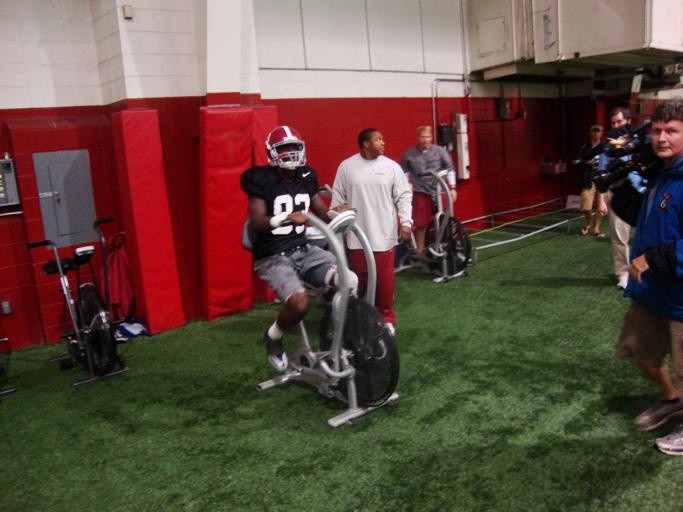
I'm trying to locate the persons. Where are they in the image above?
[325,127,415,339]
[395,125,459,256]
[575,99,683,290]
[238,126,356,372]
[614,101,683,458]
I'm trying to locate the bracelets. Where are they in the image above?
[268,212,288,228]
[449,187,457,190]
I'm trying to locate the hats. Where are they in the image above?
[590,123,603,132]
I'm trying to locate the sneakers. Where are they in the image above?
[618,274,629,290]
[263,327,290,374]
[386,321,396,337]
[656,430,683,457]
[633,392,683,431]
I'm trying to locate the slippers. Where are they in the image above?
[591,230,607,238]
[579,222,593,236]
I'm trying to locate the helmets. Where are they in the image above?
[264,124,307,169]
